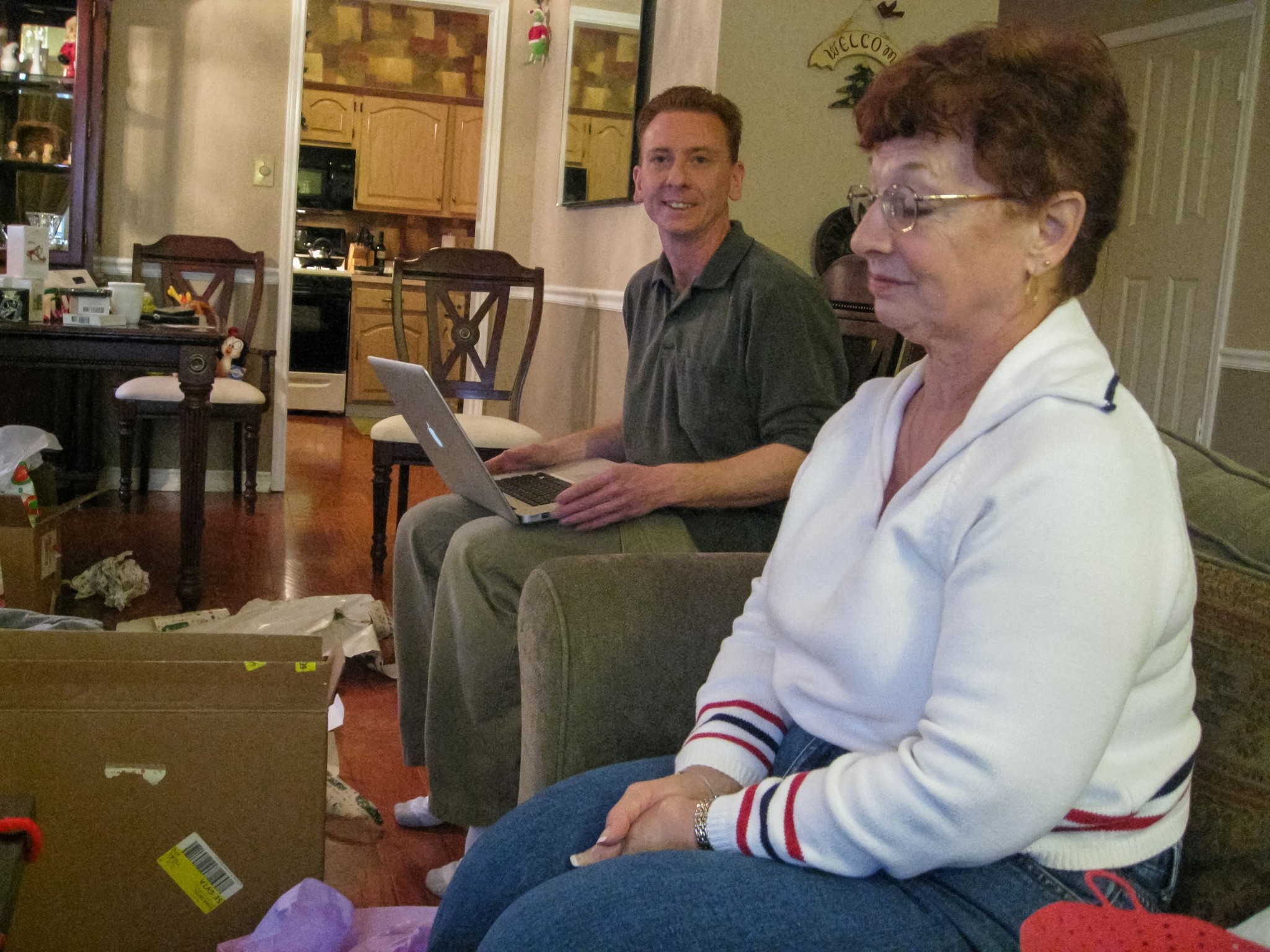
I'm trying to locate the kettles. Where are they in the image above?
[304,237,335,258]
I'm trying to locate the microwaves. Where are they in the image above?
[296,144,356,212]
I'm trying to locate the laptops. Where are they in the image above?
[366,355,578,526]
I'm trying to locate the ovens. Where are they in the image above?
[287,272,353,412]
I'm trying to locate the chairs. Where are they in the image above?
[117,235,268,515]
[367,246,546,586]
[816,252,910,394]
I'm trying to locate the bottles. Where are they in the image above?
[374,230,386,275]
[367,233,375,268]
[295,224,308,252]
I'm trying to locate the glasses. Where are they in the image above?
[847,184,1020,233]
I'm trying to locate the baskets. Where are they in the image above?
[1019,870,1270,952]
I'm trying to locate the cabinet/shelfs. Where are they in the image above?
[302,86,483,404]
[0,0,114,505]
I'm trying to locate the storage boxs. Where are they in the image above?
[1,485,109,616]
[0,625,326,952]
[0,225,125,326]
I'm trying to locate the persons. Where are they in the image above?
[58,15,78,78]
[393,85,848,896]
[426,24,1201,952]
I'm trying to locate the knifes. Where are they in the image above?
[357,225,369,247]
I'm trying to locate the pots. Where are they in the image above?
[295,254,345,267]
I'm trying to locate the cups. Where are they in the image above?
[106,281,146,325]
[26,210,64,245]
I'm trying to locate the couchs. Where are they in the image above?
[514,425,1270,952]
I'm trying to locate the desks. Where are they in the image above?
[0,319,225,608]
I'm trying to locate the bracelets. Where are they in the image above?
[695,796,716,851]
[675,769,717,799]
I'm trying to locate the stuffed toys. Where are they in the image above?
[528,5,549,66]
[217,327,248,380]
